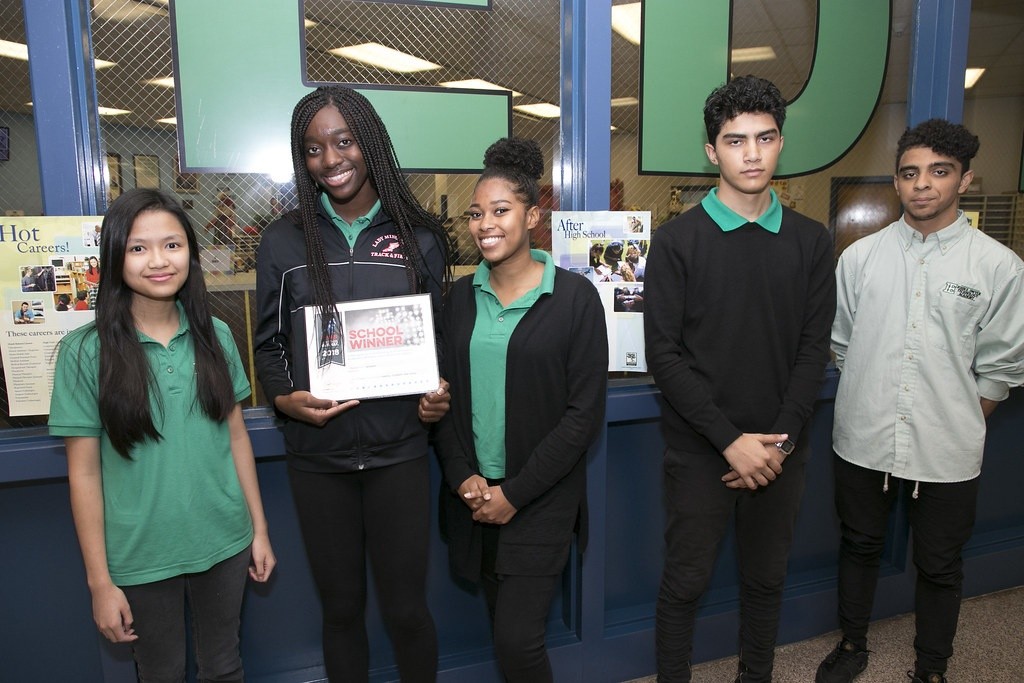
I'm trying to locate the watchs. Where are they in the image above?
[773,438,795,454]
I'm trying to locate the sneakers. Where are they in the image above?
[913,672,948,683]
[815,641,867,683]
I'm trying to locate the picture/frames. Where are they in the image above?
[173,157,199,194]
[0,126,10,161]
[107,153,123,209]
[133,154,161,191]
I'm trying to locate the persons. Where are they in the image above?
[641,74,838,683]
[13,302,34,324]
[236,195,287,236]
[55,255,101,311]
[47,186,278,683]
[813,118,1024,683]
[429,137,609,683]
[255,85,451,683]
[20,266,47,292]
[589,238,650,314]
